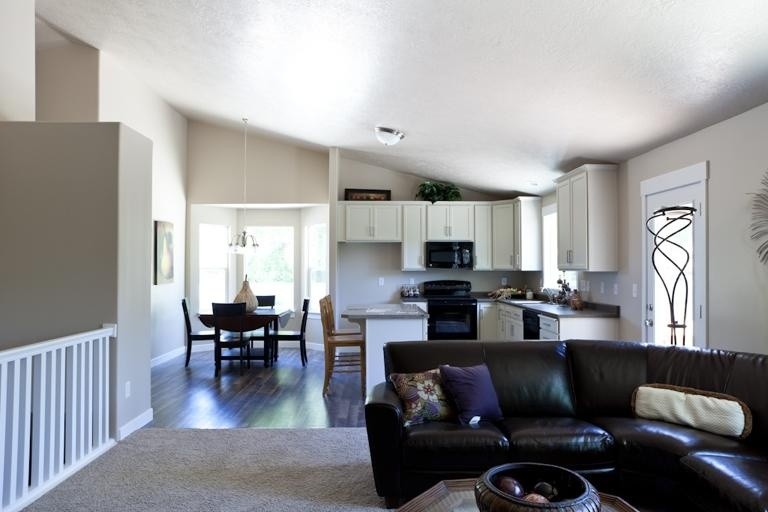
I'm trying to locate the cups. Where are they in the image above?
[526,291,533,300]
[571,299,583,310]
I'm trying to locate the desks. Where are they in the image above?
[198,308,291,370]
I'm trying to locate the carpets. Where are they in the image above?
[21,420,392,512]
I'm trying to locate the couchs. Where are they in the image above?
[361,339,766,512]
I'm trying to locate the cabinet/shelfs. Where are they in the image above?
[476,301,497,341]
[496,303,507,340]
[556,163,618,272]
[341,302,426,406]
[513,192,539,272]
[426,199,472,242]
[537,314,618,343]
[400,198,426,271]
[491,199,514,273]
[473,199,491,273]
[506,302,523,340]
[339,199,400,245]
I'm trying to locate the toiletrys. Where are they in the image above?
[572,289,581,310]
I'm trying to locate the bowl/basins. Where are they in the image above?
[473,462,601,512]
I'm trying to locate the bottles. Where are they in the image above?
[401,286,419,297]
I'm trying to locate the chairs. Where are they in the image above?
[265,298,310,366]
[324,294,367,383]
[212,302,253,375]
[316,297,361,401]
[252,296,282,362]
[182,299,216,370]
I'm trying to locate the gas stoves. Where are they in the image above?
[424,291,477,305]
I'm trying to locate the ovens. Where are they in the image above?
[428,305,477,340]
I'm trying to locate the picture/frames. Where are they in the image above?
[344,188,391,201]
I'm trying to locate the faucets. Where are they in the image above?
[543,288,552,302]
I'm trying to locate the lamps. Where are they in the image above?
[375,126,404,146]
[224,115,261,256]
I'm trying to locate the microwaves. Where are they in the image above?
[426,241,473,270]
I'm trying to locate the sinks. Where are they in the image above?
[511,300,548,304]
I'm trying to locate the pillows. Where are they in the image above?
[388,364,452,426]
[439,361,506,429]
[631,381,752,439]
